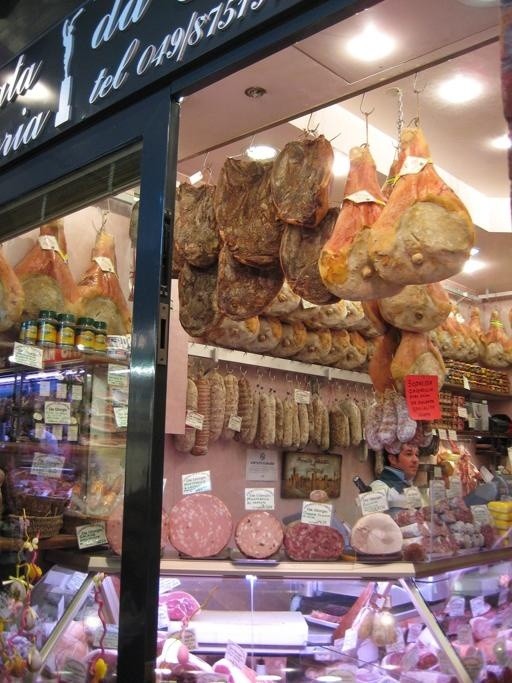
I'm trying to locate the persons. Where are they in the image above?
[378,441,422,514]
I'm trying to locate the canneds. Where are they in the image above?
[19,310,107,355]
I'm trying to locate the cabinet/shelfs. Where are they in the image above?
[0,547,512,682]
[422,382,512,438]
[0,354,130,552]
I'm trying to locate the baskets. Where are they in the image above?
[8,513,65,540]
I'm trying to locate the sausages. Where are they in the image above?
[174,373,432,476]
